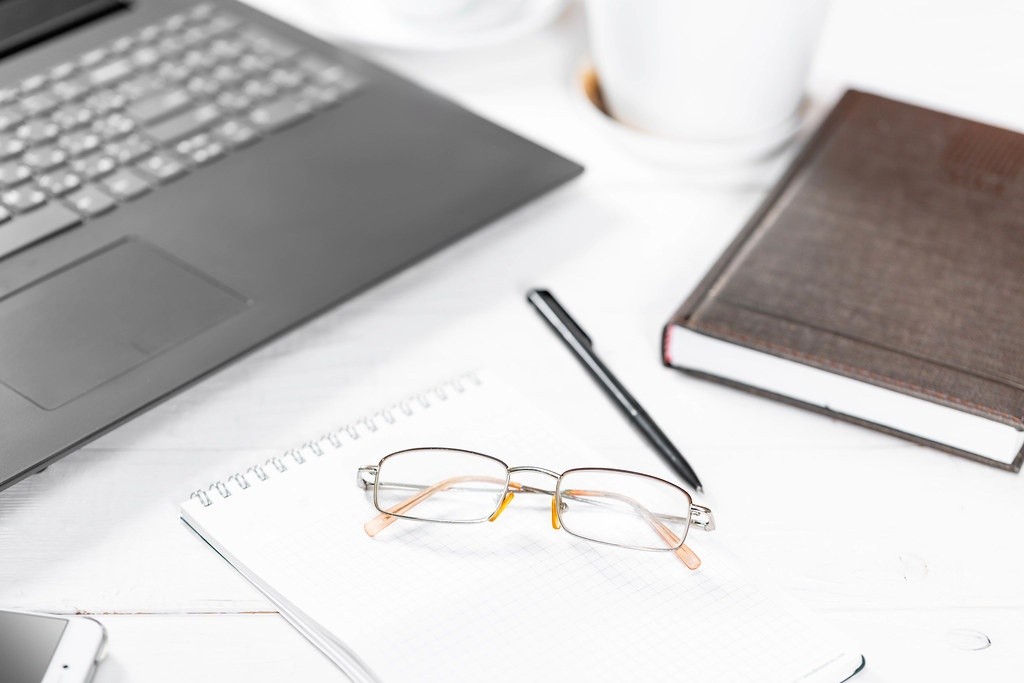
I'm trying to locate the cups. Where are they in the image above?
[588,0,828,142]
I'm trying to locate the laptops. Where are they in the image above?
[0,0,586,490]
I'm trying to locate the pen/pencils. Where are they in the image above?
[526,285,707,492]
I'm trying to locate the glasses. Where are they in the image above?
[356,447,715,570]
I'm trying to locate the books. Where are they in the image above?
[657,88,1024,473]
[176,366,868,683]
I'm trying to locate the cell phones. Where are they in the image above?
[0,608,109,682]
